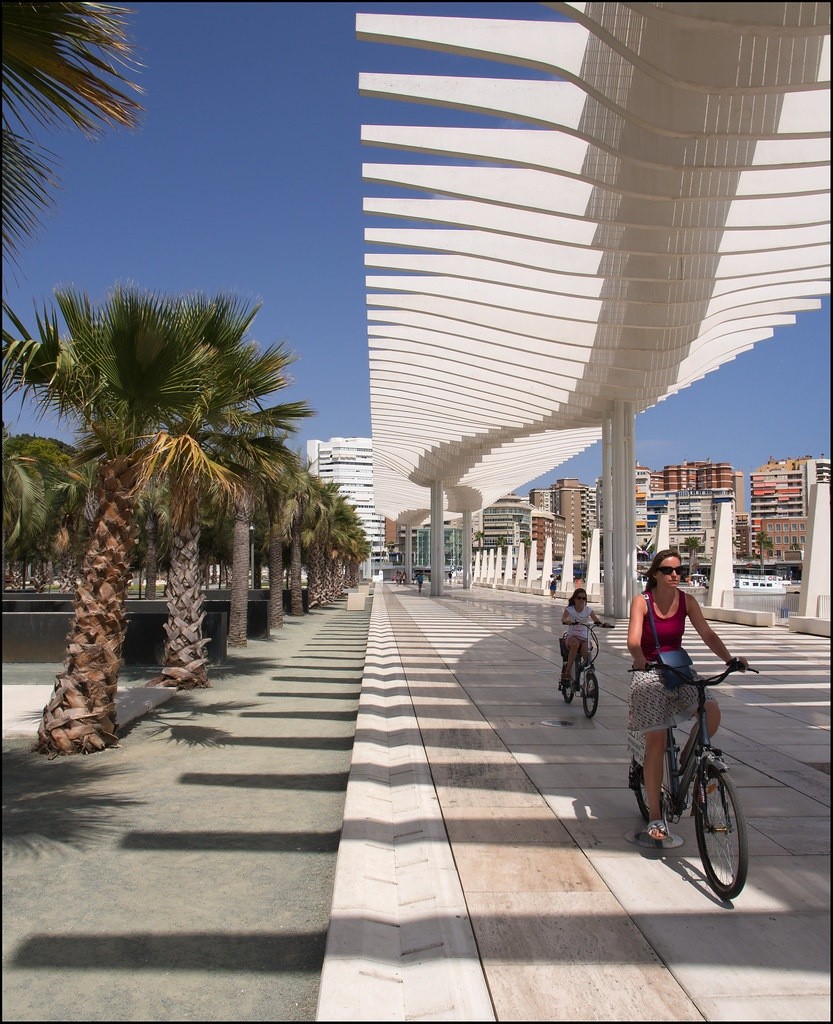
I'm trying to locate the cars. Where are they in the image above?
[553,562,587,577]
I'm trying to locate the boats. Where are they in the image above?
[732,568,791,594]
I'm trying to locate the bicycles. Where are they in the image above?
[627,661,760,899]
[558,619,615,718]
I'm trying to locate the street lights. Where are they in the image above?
[249,521,255,589]
[379,515,383,580]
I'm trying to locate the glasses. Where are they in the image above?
[576,596,586,600]
[656,566,683,575]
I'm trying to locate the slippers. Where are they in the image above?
[646,820,667,840]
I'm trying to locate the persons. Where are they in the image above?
[448,571,453,579]
[626,549,750,841]
[561,588,610,694]
[395,570,407,585]
[416,571,423,593]
[548,574,561,600]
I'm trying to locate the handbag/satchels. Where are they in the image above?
[657,649,693,689]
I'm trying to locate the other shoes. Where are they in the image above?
[562,672,571,681]
